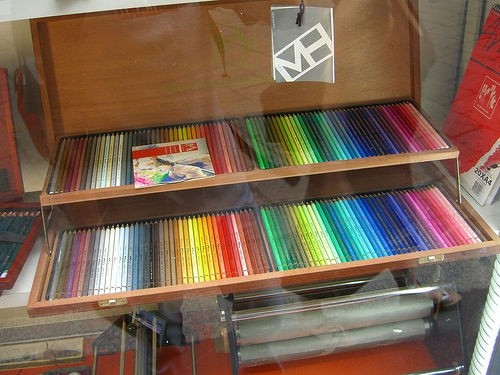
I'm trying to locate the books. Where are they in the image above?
[131,137,216,191]
[439,3,500,207]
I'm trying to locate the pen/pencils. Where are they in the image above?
[1,102,483,303]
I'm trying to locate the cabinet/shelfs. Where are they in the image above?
[2,189,500,372]
